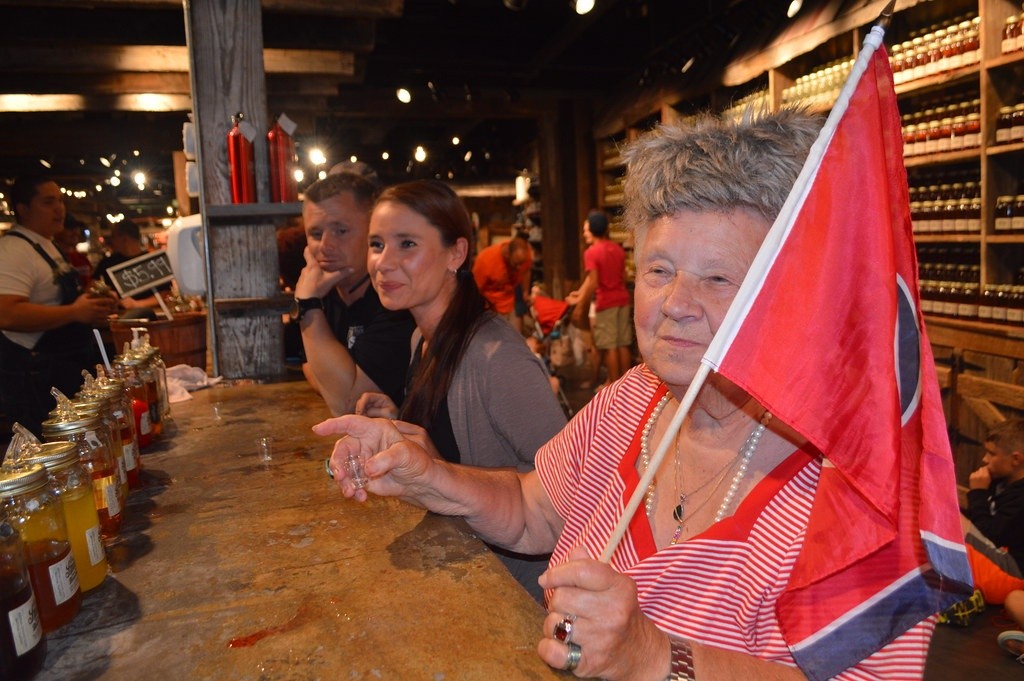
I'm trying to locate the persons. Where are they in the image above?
[275,170,636,607]
[936,418,1024,664]
[0,168,174,423]
[312,104,939,681]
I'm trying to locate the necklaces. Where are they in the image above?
[641,389,773,547]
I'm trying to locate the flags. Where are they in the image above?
[700,27,974,681]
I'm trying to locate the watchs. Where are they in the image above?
[289,297,325,324]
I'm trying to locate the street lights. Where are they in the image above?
[134,172,146,206]
[309,148,324,183]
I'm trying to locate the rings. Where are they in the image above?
[561,641,582,671]
[554,614,577,645]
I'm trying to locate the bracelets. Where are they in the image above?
[665,634,696,681]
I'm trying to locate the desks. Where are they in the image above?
[0,374,583,681]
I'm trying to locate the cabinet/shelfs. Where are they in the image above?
[594,0,1024,444]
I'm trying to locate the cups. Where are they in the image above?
[341,454,369,488]
[210,401,223,421]
[254,437,272,462]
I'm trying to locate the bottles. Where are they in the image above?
[1,346,170,681]
[267,113,299,204]
[225,112,258,205]
[550,331,563,366]
[601,11,1024,328]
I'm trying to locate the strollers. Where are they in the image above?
[516,285,580,420]
